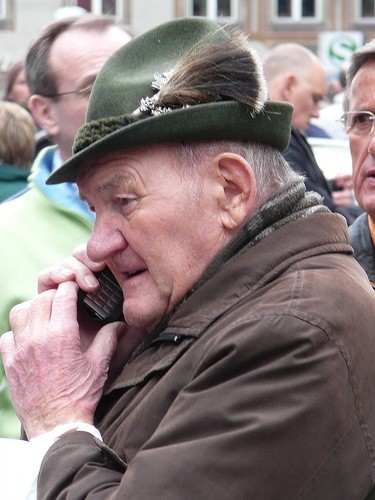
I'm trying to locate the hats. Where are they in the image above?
[45,16,294,184]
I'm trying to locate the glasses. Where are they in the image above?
[337,111,375,136]
[44,84,92,99]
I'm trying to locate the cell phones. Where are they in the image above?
[74,263,123,327]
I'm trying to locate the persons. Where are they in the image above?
[260,43,367,226]
[5,62,31,109]
[348,40,375,291]
[0,100,36,203]
[0,18,375,500]
[0,15,137,440]
